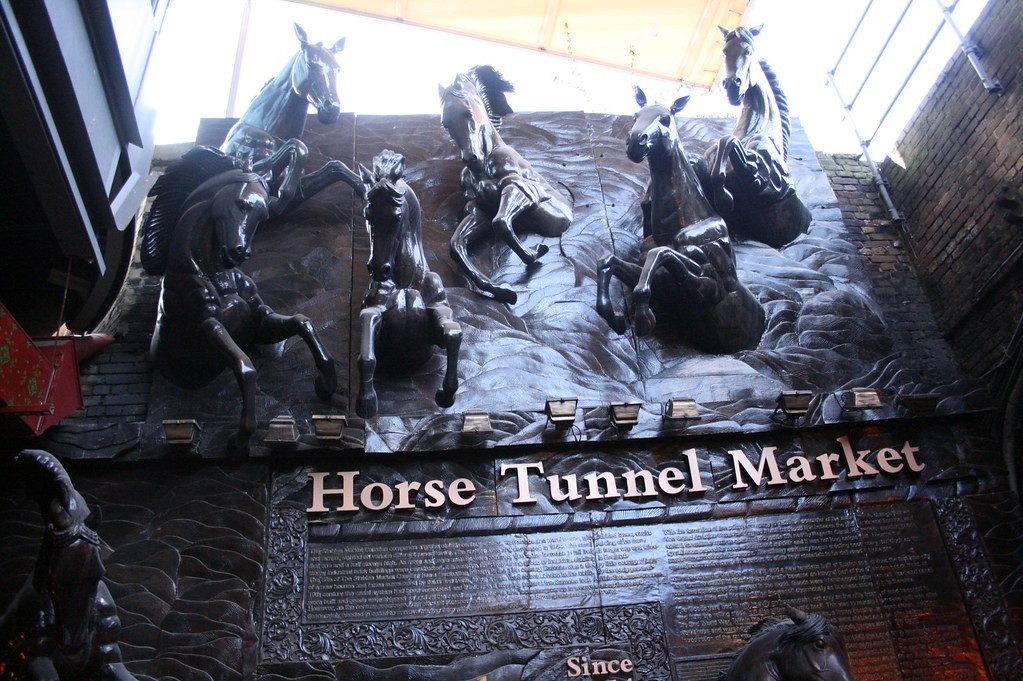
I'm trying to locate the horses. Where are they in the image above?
[139,19,462,465]
[441,62,572,307]
[594,84,767,356]
[692,19,814,251]
[715,602,854,681]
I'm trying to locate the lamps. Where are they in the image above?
[460,412,493,434]
[312,414,347,440]
[263,415,299,445]
[844,387,881,412]
[661,398,701,422]
[161,419,200,444]
[543,397,578,430]
[608,402,643,428]
[772,390,813,419]
